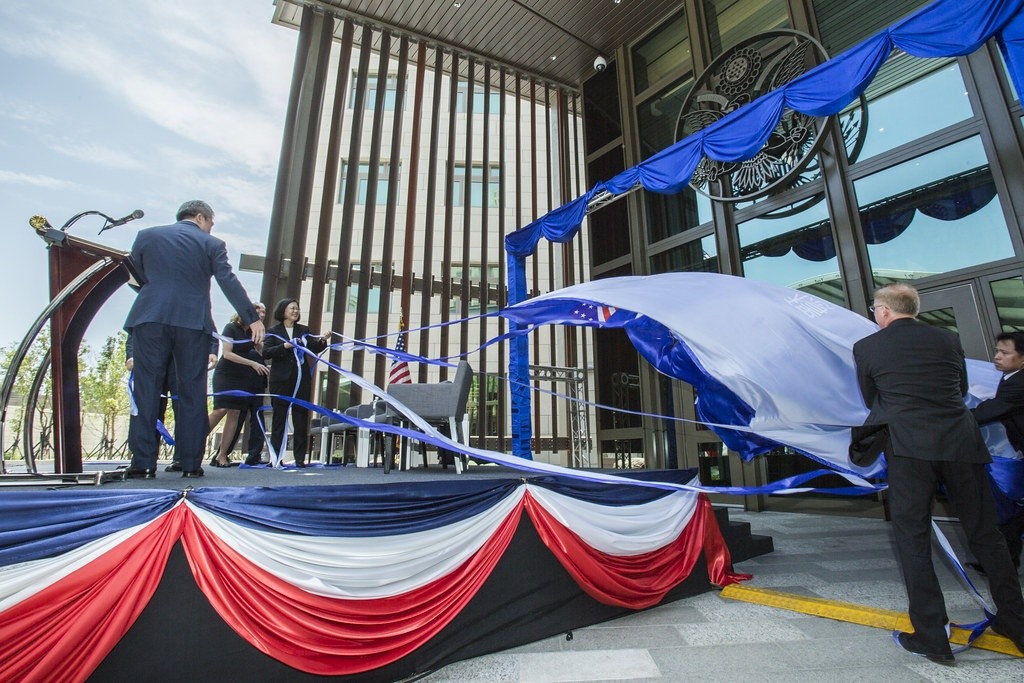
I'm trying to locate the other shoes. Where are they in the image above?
[965,562,987,574]
[211,456,241,466]
[216,459,231,467]
[267,460,284,467]
[245,459,269,466]
[295,460,306,468]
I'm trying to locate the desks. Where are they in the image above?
[398,414,470,470]
[357,415,376,468]
[320,426,346,466]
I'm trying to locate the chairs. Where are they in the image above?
[308,361,475,474]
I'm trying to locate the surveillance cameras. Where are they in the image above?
[593,57,607,73]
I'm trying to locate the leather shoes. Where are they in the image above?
[182,466,204,477]
[125,463,156,478]
[165,461,182,472]
[991,617,1024,653]
[899,632,955,663]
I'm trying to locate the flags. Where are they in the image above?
[390,327,413,385]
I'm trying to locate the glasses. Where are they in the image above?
[870,305,889,312]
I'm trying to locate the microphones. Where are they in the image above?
[104,210,144,231]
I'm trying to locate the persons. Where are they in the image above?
[849,283,1024,662]
[967,326,1024,577]
[125,296,332,472]
[122,200,266,483]
